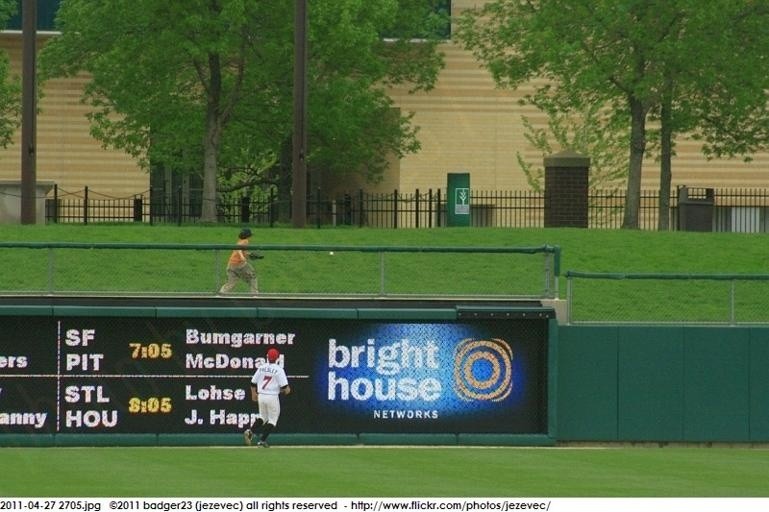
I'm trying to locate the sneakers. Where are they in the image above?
[257,441,268,448]
[244,429,253,445]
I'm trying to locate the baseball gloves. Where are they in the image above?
[249,252,264,261]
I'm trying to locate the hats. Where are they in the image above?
[240,228,251,238]
[269,348,279,360]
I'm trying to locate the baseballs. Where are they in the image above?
[329,251,334,255]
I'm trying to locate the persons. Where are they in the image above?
[243,347,292,448]
[217,229,265,293]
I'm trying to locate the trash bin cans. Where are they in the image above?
[678,187,715,232]
